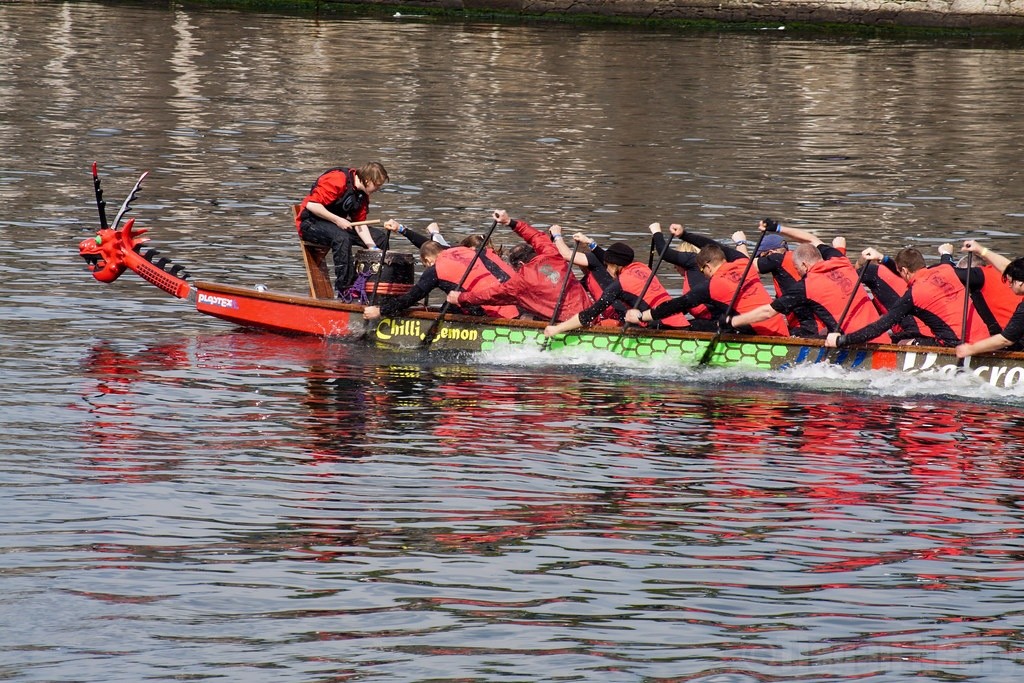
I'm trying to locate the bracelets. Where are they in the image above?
[776,225,780,232]
[588,241,597,250]
[399,225,403,232]
[553,234,560,237]
[882,255,888,263]
[736,240,746,245]
[979,248,987,259]
[373,245,378,250]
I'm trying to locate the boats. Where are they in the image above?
[80,160,1024,396]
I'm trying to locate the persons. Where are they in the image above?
[364,210,1024,360]
[298,161,388,298]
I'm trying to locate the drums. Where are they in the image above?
[355,249,418,306]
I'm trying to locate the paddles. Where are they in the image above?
[609,230,674,350]
[646,236,658,269]
[695,230,766,367]
[819,256,872,355]
[958,243,974,369]
[540,240,578,353]
[414,212,500,349]
[356,226,392,337]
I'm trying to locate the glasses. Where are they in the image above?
[372,181,381,190]
[699,260,711,273]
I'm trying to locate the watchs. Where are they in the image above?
[637,311,643,321]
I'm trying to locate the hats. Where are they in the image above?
[754,234,788,257]
[603,242,634,266]
